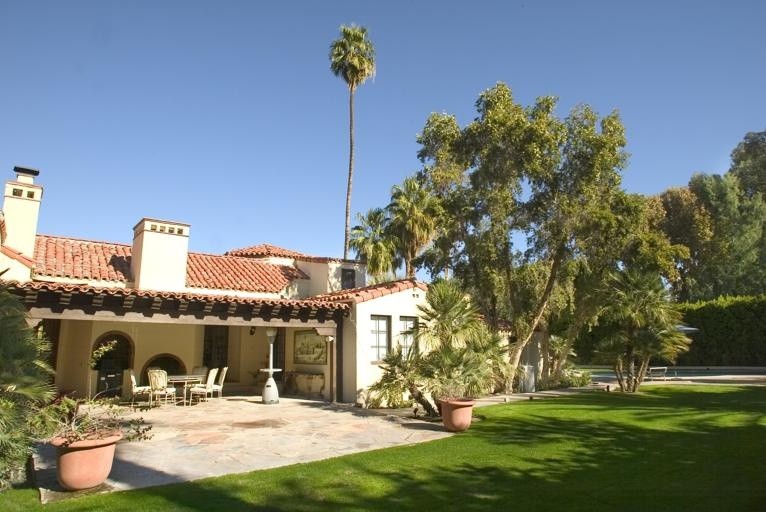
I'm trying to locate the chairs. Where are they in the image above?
[129,366,229,409]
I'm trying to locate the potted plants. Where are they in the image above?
[424,369,487,432]
[41,384,162,492]
